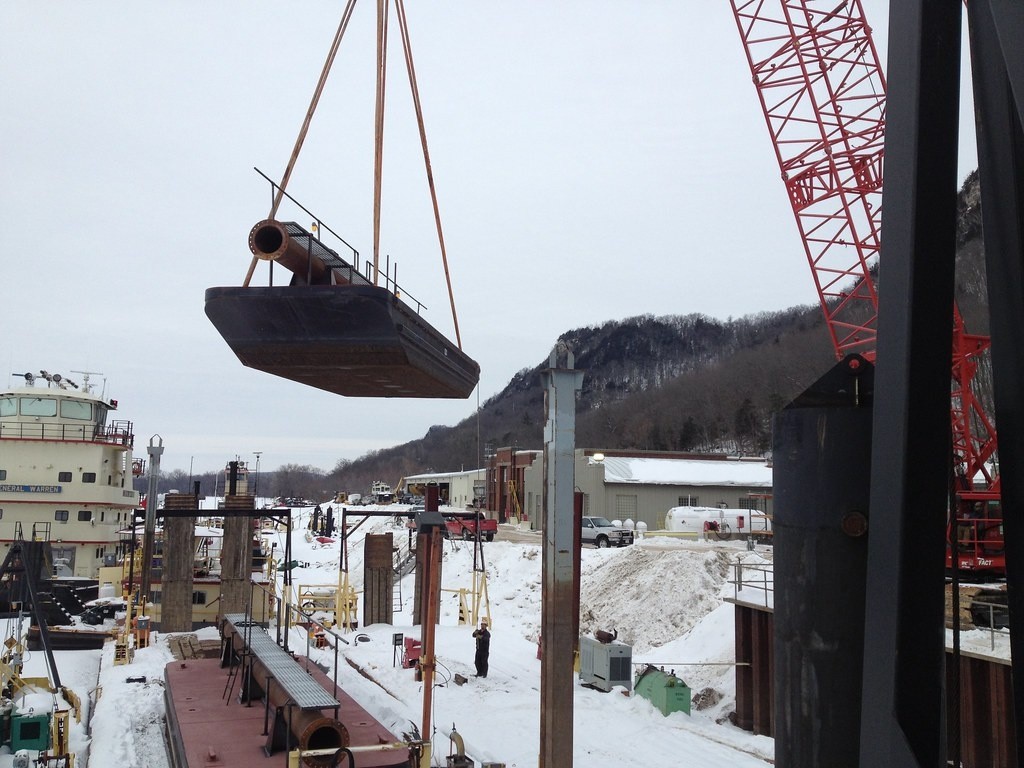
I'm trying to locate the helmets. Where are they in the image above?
[481,620,488,626]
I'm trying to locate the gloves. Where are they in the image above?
[474,629,478,634]
[476,634,483,640]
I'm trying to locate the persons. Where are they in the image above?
[473,621,490,678]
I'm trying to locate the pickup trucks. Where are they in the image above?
[443,512,498,542]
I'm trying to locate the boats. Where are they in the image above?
[0,368,145,576]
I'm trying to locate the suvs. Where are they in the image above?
[580,515,635,549]
